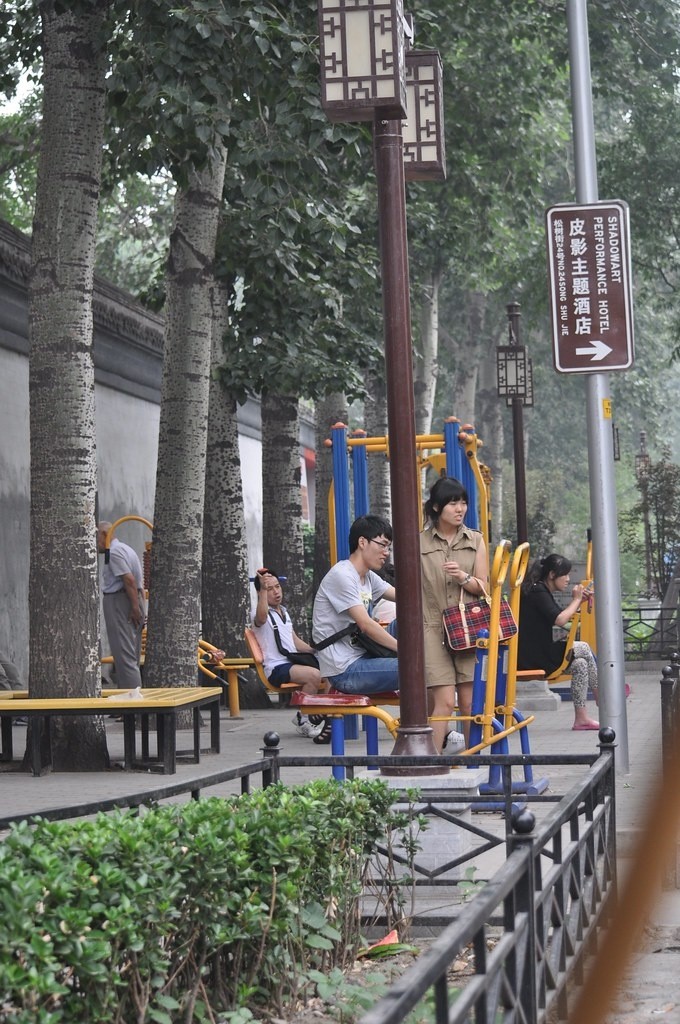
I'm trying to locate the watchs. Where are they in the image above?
[458,573,471,585]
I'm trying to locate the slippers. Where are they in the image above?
[572,719,600,729]
[625,683,630,697]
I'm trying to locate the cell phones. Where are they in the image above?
[585,581,593,591]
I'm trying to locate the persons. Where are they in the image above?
[420,477,487,755]
[97,521,146,722]
[308,515,484,754]
[517,554,631,729]
[250,571,342,737]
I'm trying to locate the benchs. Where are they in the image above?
[0,687,224,774]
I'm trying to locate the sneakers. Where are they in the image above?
[442,731,483,754]
[292,714,300,725]
[296,717,321,737]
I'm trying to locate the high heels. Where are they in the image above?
[314,720,331,743]
[308,713,327,725]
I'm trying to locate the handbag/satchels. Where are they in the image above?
[442,577,518,654]
[354,629,393,658]
[287,652,320,670]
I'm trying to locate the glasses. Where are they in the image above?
[365,536,392,553]
